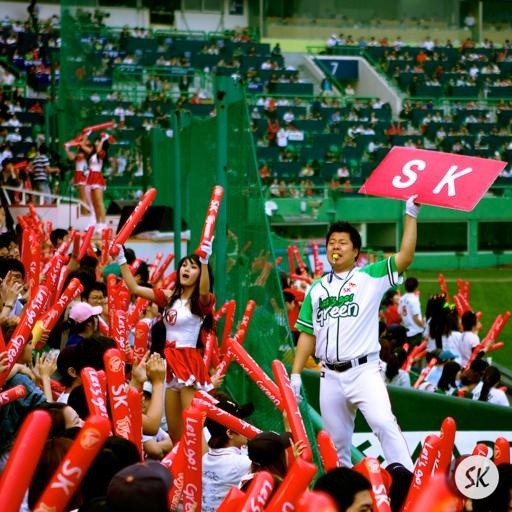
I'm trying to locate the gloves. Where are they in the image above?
[288,374,304,403]
[65,130,110,151]
[322,357,370,371]
[199,235,215,264]
[109,239,127,265]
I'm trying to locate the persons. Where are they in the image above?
[1,3,510,511]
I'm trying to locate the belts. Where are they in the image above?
[405,194,421,218]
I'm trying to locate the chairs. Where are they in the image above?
[0,17,512,196]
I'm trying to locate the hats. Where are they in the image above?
[206,399,254,434]
[106,462,171,501]
[69,301,102,324]
[103,264,120,280]
[439,350,459,362]
[388,324,409,334]
[247,432,293,463]
